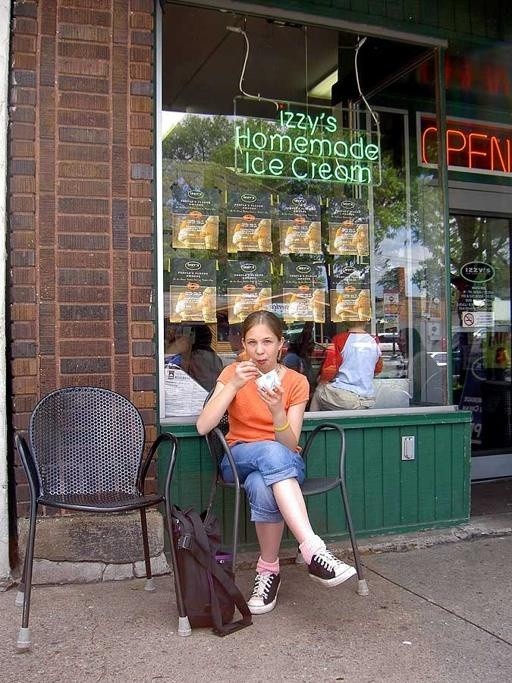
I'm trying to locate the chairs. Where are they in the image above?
[202,387,369,597]
[14,384,192,651]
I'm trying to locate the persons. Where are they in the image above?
[395,326,444,406]
[165,308,384,410]
[193,308,360,616]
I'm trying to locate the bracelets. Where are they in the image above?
[272,421,292,433]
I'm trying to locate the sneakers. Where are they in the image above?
[247,572,281,614]
[307,550,357,586]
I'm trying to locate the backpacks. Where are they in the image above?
[170,503,253,638]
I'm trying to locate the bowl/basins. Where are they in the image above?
[254,369,280,392]
